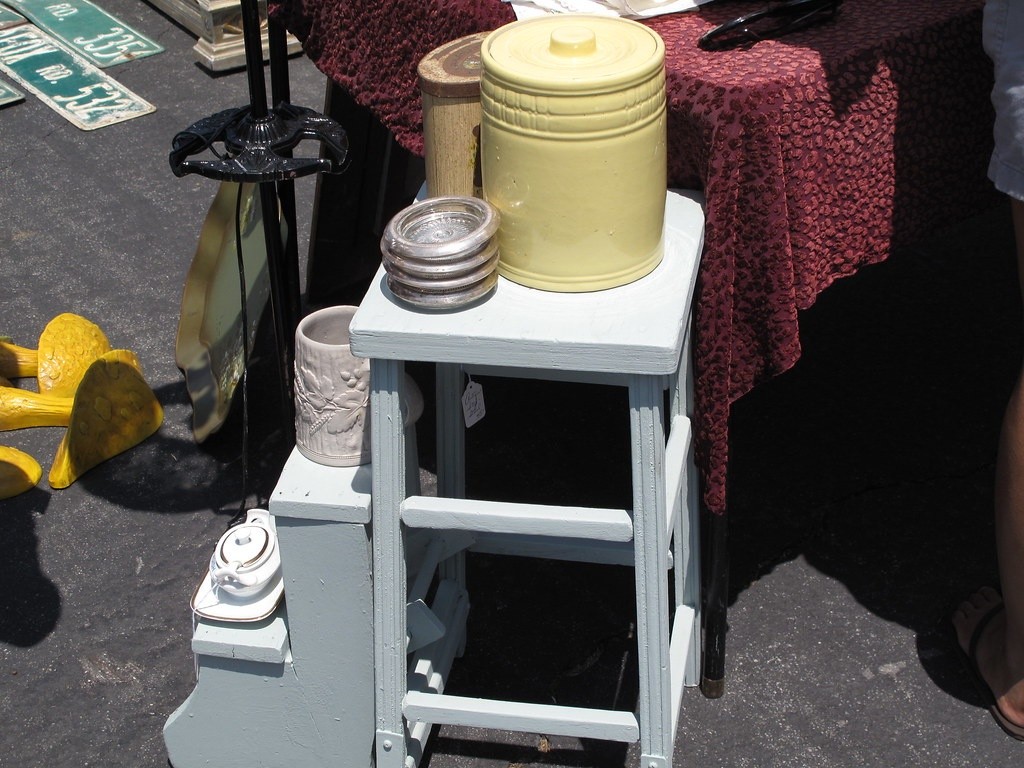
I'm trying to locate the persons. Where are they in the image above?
[950,1,1024,742]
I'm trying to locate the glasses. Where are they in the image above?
[701,0,846,51]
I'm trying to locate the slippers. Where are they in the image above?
[952,598,1024,741]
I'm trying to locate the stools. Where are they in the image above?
[348,190,707,767]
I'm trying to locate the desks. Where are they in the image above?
[240,0,995,698]
